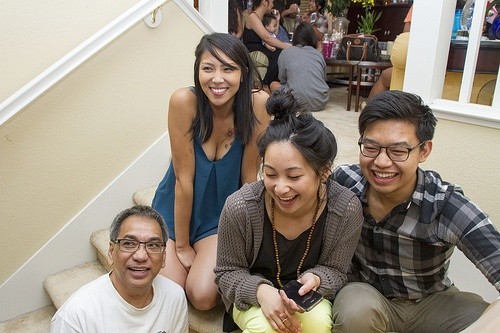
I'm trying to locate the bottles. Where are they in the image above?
[323,32,344,59]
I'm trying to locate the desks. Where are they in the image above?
[322,57,392,113]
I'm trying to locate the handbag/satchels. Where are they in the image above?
[336,32,381,66]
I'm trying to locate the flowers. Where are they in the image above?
[352,0,381,34]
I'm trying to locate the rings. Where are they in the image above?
[282,317,289,323]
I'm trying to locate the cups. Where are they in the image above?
[322,41,333,57]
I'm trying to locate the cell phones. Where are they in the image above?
[279,280,324,312]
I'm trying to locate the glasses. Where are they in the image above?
[357,132,426,162]
[111,238,166,254]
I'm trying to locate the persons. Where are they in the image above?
[270,21,332,112]
[325,88,500,332]
[50,203,189,333]
[152,32,280,315]
[229,0,338,84]
[357,4,411,110]
[214,91,364,333]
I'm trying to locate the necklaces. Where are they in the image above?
[269,194,323,289]
